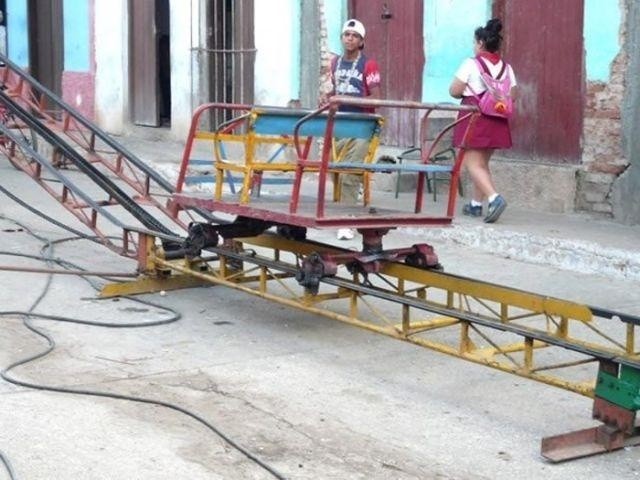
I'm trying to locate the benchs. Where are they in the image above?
[176,100,480,222]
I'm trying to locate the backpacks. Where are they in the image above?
[474,56,515,118]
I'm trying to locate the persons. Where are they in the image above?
[448,18,519,222]
[318,17,383,240]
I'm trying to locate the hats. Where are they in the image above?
[342,19,366,51]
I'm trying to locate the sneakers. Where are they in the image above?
[483,194,508,223]
[336,213,356,240]
[462,200,483,217]
